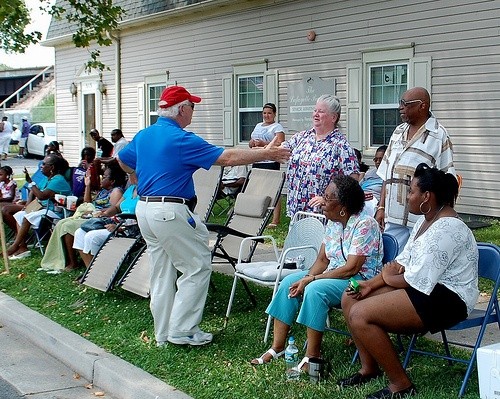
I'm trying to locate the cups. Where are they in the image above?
[67,196,78,210]
[310,357,324,376]
[54,194,66,212]
[96,148,103,158]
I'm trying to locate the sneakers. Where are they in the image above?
[167,330,212,345]
[157,341,168,347]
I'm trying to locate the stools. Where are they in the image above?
[213,186,242,217]
[26,214,53,256]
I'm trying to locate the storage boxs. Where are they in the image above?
[476,343,500,399]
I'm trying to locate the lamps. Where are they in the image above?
[99,80,107,100]
[70,83,77,101]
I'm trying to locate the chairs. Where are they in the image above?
[302,233,399,363]
[117,164,224,298]
[210,167,286,306]
[79,214,145,293]
[403,242,500,399]
[220,210,326,342]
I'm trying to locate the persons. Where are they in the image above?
[217,164,248,199]
[0,116,140,274]
[375,86,457,255]
[337,163,479,399]
[115,86,293,346]
[352,145,389,218]
[267,94,360,234]
[249,103,286,229]
[251,173,384,376]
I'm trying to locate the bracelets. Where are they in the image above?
[377,207,385,210]
[28,182,36,188]
[305,275,315,280]
[126,169,135,175]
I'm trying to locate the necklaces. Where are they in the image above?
[413,203,446,240]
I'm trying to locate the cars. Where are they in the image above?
[22,122,56,158]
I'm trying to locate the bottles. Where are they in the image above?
[285,337,301,381]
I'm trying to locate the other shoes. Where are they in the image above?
[64,265,78,272]
[8,250,31,260]
[266,223,279,230]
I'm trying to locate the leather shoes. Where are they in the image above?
[366,383,417,399]
[337,369,382,388]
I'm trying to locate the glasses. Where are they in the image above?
[417,163,434,175]
[41,161,56,165]
[179,103,194,110]
[321,191,339,204]
[373,157,382,162]
[399,100,422,107]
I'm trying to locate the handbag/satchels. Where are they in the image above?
[25,199,42,214]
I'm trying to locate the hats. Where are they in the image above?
[111,129,123,137]
[3,115,8,118]
[21,116,27,120]
[89,129,98,134]
[12,124,18,128]
[159,86,201,108]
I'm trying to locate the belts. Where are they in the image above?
[140,197,190,205]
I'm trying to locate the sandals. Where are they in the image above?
[251,348,285,366]
[288,356,322,375]
